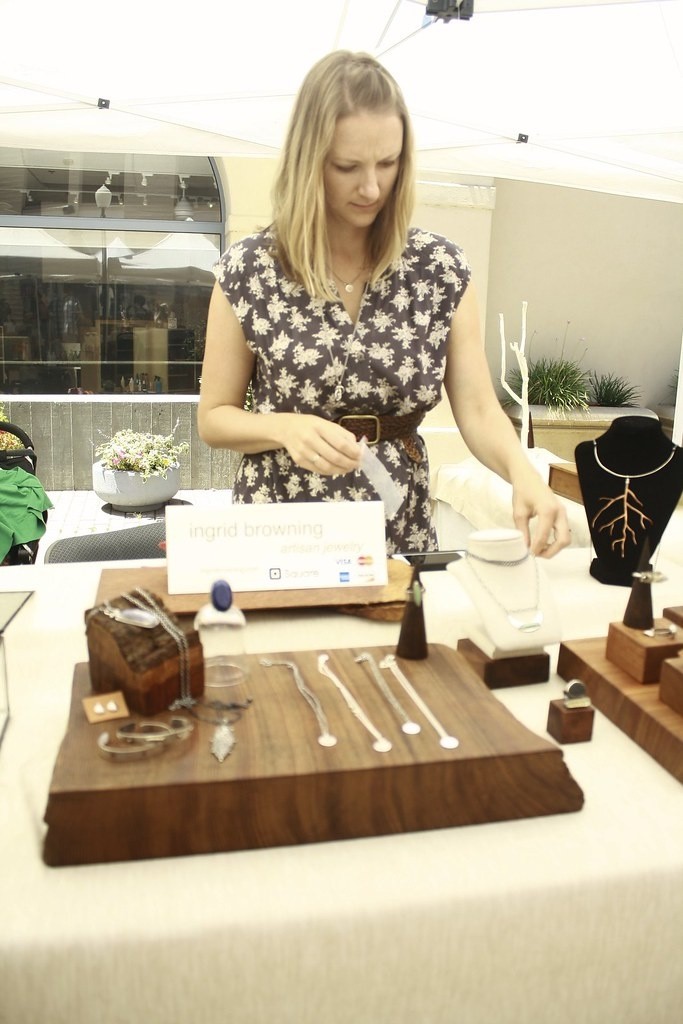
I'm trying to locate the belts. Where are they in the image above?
[333,411,429,445]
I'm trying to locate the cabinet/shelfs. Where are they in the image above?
[131,326,198,394]
[81,319,164,394]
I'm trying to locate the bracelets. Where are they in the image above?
[117,714,194,743]
[90,721,176,763]
[200,660,247,688]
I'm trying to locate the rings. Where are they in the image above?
[194,579,246,633]
[310,454,320,464]
[648,626,674,638]
[631,569,667,584]
[406,580,426,607]
[565,681,587,701]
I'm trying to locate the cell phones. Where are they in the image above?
[390,549,467,573]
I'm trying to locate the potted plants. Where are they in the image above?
[91,415,190,512]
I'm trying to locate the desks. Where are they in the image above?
[0,541,683,1024]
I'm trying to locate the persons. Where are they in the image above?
[126,296,155,320]
[193,48,573,558]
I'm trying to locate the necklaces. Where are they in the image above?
[464,544,541,636]
[315,655,392,755]
[593,438,679,559]
[325,258,366,295]
[99,597,161,629]
[261,658,337,750]
[355,652,421,736]
[188,696,252,764]
[378,654,458,750]
[121,587,198,711]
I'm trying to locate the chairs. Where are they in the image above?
[44,519,166,564]
